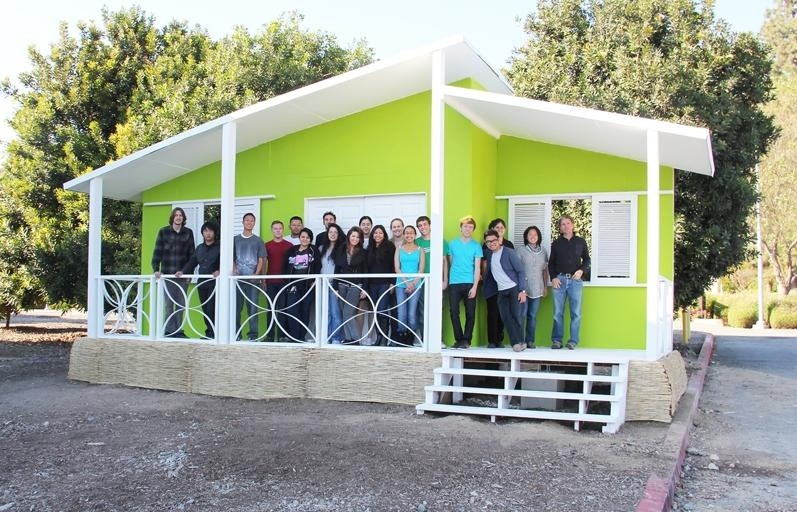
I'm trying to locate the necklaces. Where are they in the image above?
[531,245,537,247]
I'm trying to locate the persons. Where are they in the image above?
[481,218,514,348]
[173,221,220,339]
[547,215,591,350]
[151,206,195,338]
[482,230,528,351]
[447,216,484,350]
[233,211,451,349]
[513,226,550,348]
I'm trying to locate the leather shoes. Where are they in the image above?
[379,334,387,346]
[164,331,175,337]
[175,332,188,338]
[371,335,381,346]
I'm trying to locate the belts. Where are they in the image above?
[560,273,572,279]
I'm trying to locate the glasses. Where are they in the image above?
[486,238,498,245]
[291,224,301,227]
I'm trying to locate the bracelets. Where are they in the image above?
[519,290,528,294]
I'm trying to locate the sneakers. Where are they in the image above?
[450,343,458,349]
[330,338,370,346]
[551,340,563,349]
[388,330,445,349]
[512,341,535,352]
[565,342,575,350]
[497,343,505,348]
[457,341,470,350]
[488,343,496,348]
[248,335,306,342]
